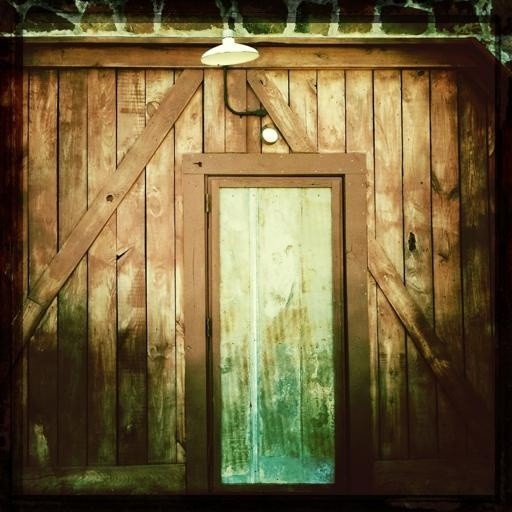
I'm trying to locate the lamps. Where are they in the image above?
[199,2,268,117]
